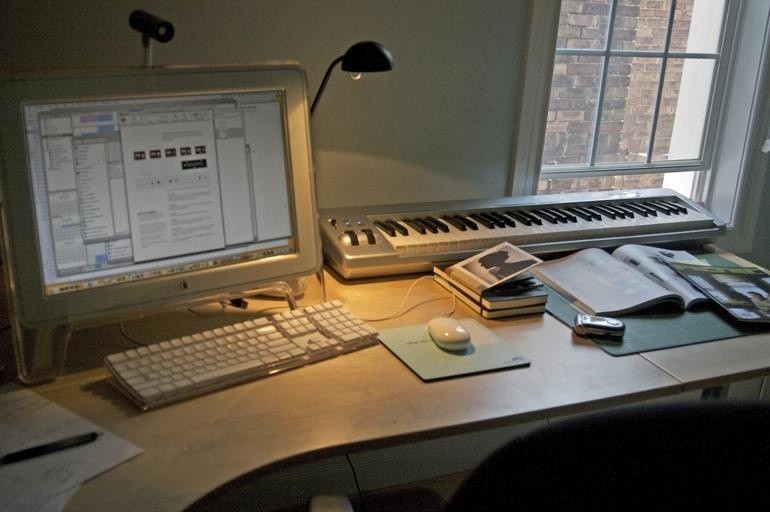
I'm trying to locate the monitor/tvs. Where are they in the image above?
[0,60,323,387]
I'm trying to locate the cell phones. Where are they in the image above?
[576,313,625,339]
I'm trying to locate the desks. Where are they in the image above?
[2,245,770,511]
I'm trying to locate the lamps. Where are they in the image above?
[307,40,398,122]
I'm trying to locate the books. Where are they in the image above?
[432,240,549,320]
[667,262,770,323]
[531,244,711,317]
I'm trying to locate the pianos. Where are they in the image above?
[317,187,727,280]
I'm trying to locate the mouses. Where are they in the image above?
[429,317,470,350]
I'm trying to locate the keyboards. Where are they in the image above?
[102,300,379,411]
